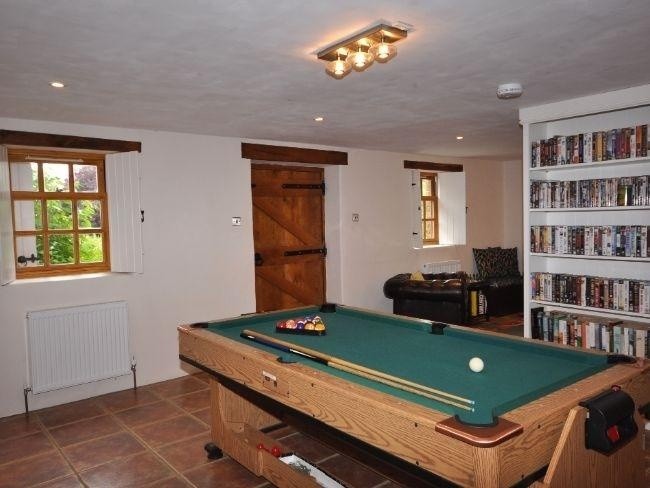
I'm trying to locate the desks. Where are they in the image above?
[176,302,649,487]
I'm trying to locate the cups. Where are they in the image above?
[618,184,633,207]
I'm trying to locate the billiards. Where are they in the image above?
[469,357,484,372]
[276,315,325,331]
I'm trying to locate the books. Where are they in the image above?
[530,124,650,360]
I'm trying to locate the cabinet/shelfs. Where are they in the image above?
[518,84,650,359]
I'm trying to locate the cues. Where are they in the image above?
[240,328,475,412]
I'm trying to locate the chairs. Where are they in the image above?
[383,271,470,325]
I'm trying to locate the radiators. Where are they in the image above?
[421,260,462,275]
[23,299,140,422]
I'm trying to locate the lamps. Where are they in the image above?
[315,22,413,81]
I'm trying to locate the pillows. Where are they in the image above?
[472,246,519,279]
